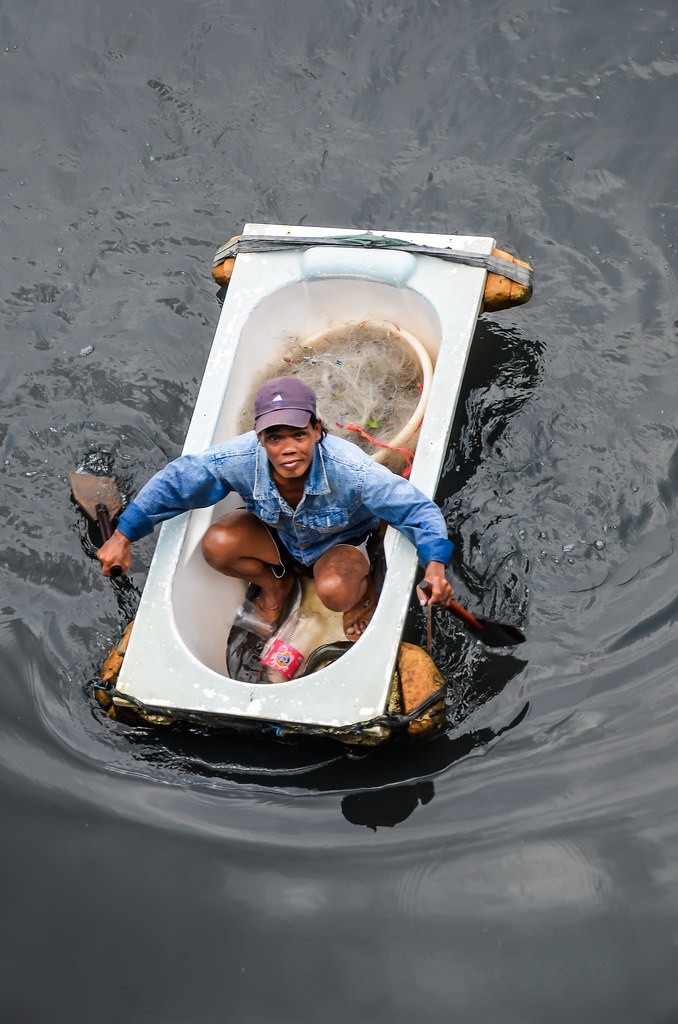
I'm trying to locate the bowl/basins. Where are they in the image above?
[234,318,433,476]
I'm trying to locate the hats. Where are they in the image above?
[254,377,316,435]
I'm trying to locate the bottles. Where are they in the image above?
[257,605,330,687]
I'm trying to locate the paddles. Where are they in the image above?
[418,580,528,648]
[66,471,123,578]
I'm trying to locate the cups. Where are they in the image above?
[227,597,277,640]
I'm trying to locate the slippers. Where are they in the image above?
[342,573,377,642]
[250,572,297,638]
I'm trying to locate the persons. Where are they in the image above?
[95,377,456,641]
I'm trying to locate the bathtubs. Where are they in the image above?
[110,223,498,740]
